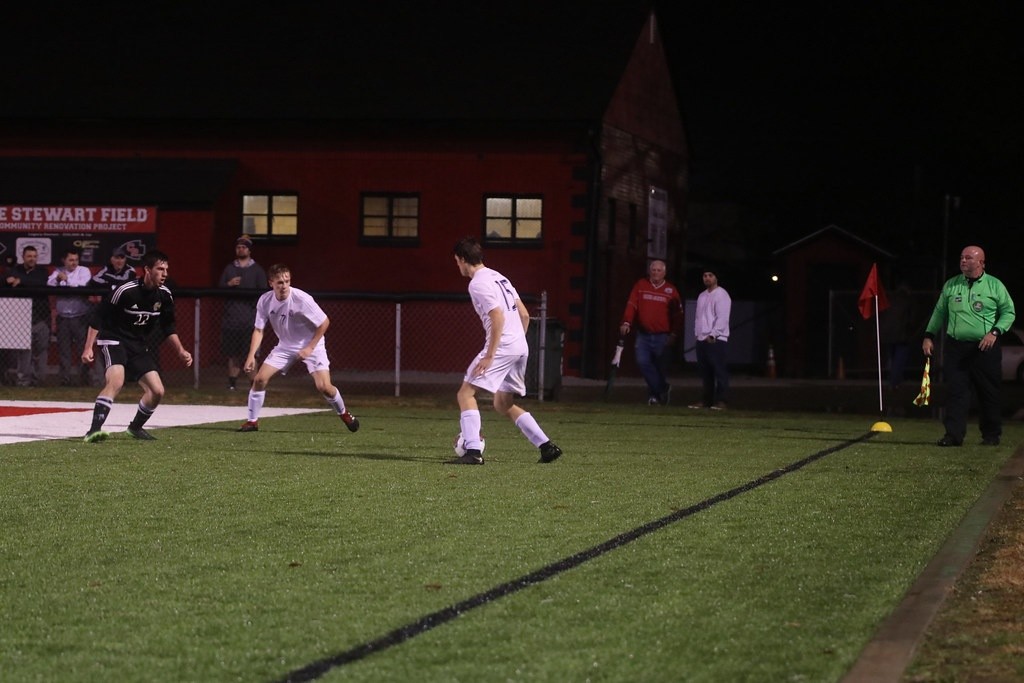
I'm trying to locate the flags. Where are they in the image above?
[857,264,891,320]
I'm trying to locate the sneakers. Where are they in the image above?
[236,421,258,432]
[125,421,157,439]
[84,432,108,443]
[339,410,359,432]
[443,449,484,465]
[538,441,562,463]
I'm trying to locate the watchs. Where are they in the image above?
[991,330,998,336]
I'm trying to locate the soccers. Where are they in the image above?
[453,431,485,458]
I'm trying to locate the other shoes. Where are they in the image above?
[660,384,675,408]
[710,402,727,411]
[938,434,963,446]
[979,436,1000,445]
[687,402,704,409]
[648,396,658,407]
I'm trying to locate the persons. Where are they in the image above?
[80,250,192,441]
[621,259,683,405]
[90,249,137,289]
[0,245,50,389]
[217,234,265,393]
[238,264,360,434]
[923,246,1016,447]
[46,250,94,386]
[688,266,732,411]
[443,237,563,465]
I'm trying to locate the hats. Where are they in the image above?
[112,248,125,257]
[236,237,252,248]
[702,266,720,280]
